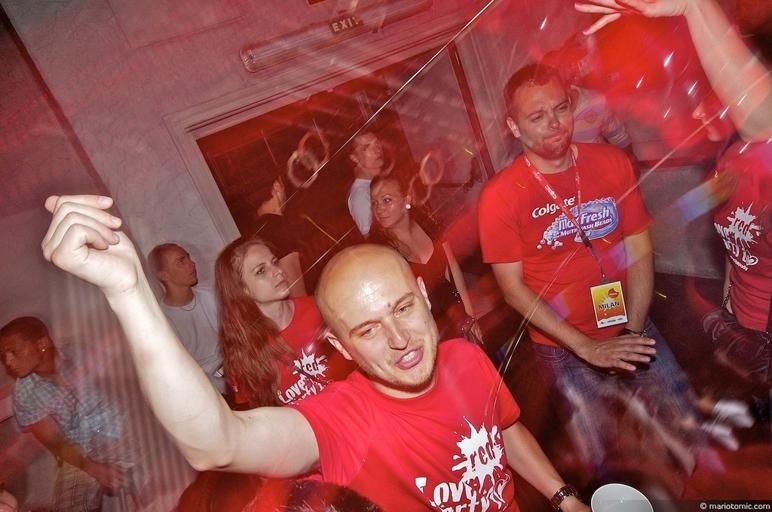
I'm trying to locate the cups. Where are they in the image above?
[591,483,654,512]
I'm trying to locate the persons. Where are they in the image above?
[574,0,772,333]
[0,130,592,512]
[543,51,640,180]
[478,64,710,479]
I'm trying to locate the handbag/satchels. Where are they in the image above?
[702,319,772,390]
